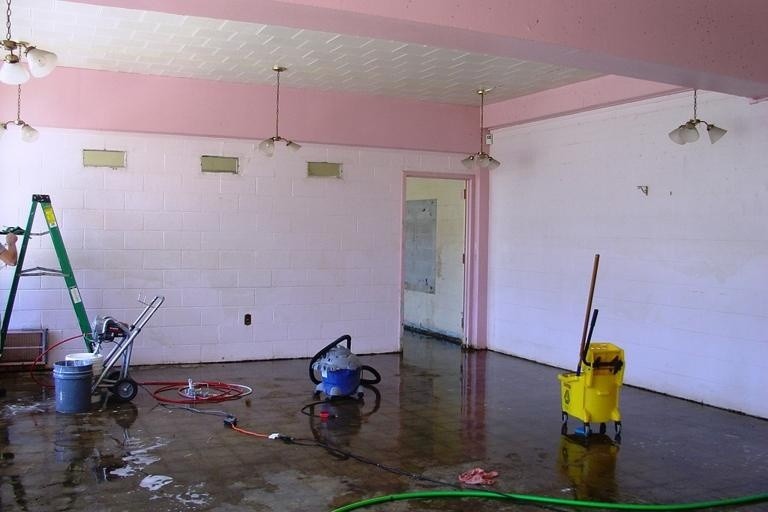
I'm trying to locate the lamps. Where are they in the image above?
[0,0,59,86]
[1,86,41,143]
[669,88,728,144]
[258,67,302,158]
[460,88,501,171]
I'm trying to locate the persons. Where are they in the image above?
[0,233,17,266]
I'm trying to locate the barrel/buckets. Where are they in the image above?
[53,359,91,411]
[64,353,104,404]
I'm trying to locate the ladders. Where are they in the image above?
[0,194,95,359]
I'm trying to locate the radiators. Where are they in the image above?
[1,330,49,365]
